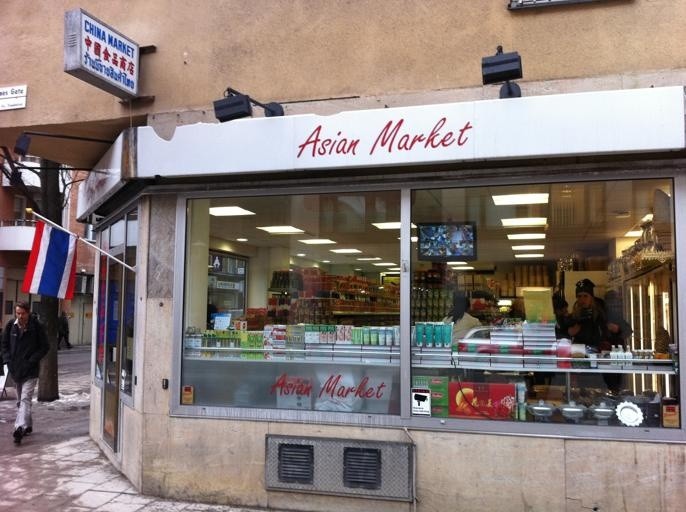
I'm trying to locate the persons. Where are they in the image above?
[442,295,484,342]
[553,278,631,395]
[0,299,50,444]
[56,311,74,350]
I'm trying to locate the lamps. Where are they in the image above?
[480,45,523,99]
[211,84,287,123]
[13,128,118,158]
[10,164,95,186]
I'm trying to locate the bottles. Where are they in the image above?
[610,345,633,366]
[648,401,660,426]
[184,326,203,358]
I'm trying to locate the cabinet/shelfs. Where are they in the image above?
[181,248,680,429]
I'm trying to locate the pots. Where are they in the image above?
[557,401,589,419]
[590,403,615,419]
[525,399,557,417]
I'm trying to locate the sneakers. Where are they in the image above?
[13,426,32,442]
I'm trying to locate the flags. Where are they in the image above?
[20,218,79,301]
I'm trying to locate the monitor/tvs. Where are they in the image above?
[416,220,477,263]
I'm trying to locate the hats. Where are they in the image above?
[576,279,595,298]
